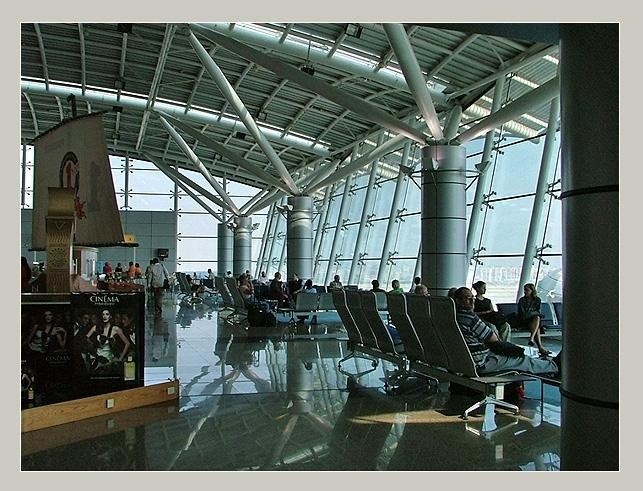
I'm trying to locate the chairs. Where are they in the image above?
[374,291,387,311]
[279,292,319,334]
[317,292,338,311]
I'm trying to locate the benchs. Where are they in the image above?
[332,289,409,388]
[496,302,563,337]
[386,292,537,420]
[175,272,202,301]
[214,276,256,323]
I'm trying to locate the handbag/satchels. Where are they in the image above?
[490,341,525,357]
[164,280,169,289]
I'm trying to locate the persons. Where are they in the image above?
[414,284,429,297]
[291,324,320,371]
[101,257,171,322]
[187,269,318,324]
[147,318,170,363]
[453,287,563,375]
[472,280,511,341]
[388,279,404,293]
[271,334,284,352]
[447,287,457,300]
[21,308,73,371]
[518,282,550,355]
[369,279,386,292]
[21,256,33,294]
[409,277,422,293]
[73,307,136,377]
[330,274,343,288]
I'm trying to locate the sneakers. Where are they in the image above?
[539,351,552,354]
[528,341,534,345]
